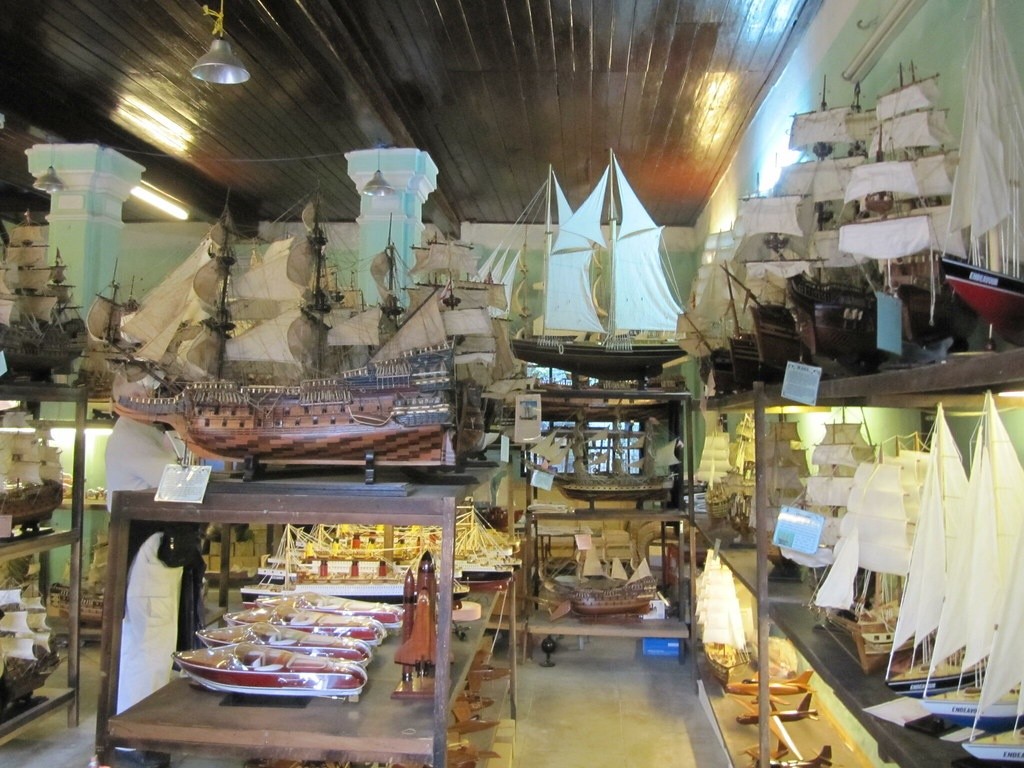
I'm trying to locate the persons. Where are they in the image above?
[105,414,205,768]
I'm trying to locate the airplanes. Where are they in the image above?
[726,666,816,706]
[743,740,833,768]
[735,695,819,759]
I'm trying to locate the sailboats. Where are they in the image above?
[694,545,751,677]
[706,390,1024,764]
[541,529,659,617]
[0,139,515,767]
[695,0,1024,387]
[469,146,690,379]
[523,411,684,502]
